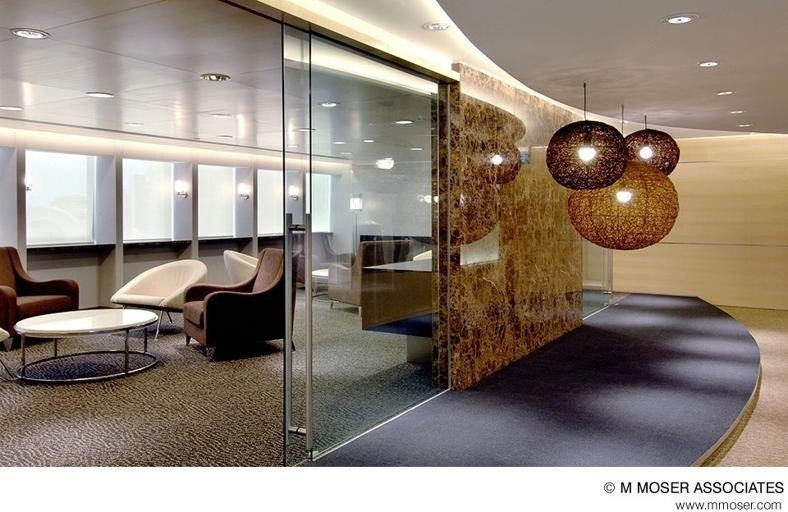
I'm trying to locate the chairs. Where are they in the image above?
[0,234,411,362]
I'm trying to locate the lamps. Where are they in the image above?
[543,82,680,252]
